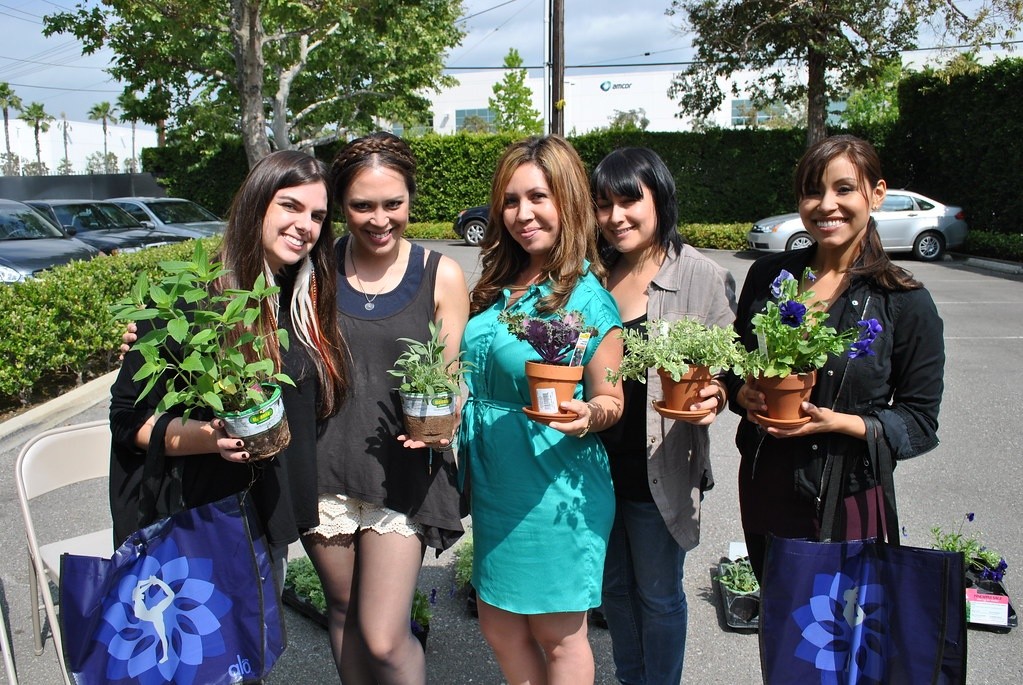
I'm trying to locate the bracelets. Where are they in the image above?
[577,418,593,438]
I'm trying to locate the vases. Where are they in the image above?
[747,367,817,420]
[958,558,1019,630]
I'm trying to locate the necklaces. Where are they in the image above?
[350,237,398,310]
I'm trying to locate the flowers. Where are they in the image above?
[929,515,1006,581]
[743,267,881,379]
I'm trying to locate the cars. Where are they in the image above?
[19,199,195,260]
[103,196,230,242]
[452,203,491,247]
[0,198,106,288]
[745,188,970,263]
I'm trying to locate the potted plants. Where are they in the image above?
[284,554,432,654]
[387,320,479,445]
[105,239,297,458]
[604,317,746,411]
[498,309,599,414]
[718,553,761,630]
[452,538,477,615]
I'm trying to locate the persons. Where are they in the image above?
[111,149,350,592]
[456,134,625,685]
[727,134,946,586]
[119,131,469,685]
[591,146,738,685]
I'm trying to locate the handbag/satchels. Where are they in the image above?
[759,415,967,685]
[58,413,287,685]
